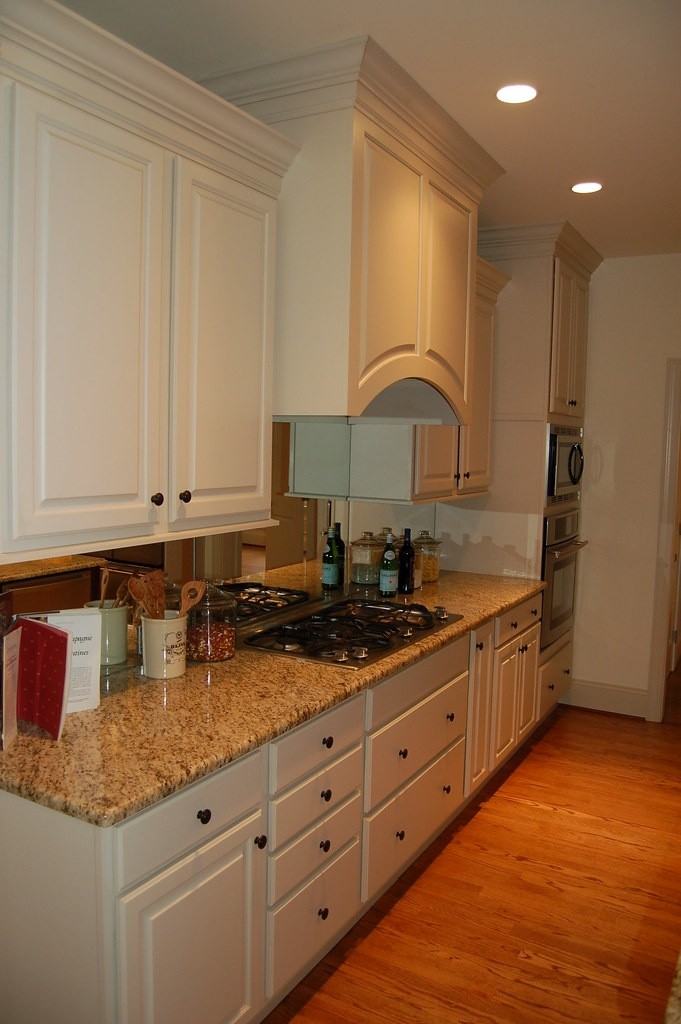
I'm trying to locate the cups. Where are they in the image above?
[84,599,128,664]
[140,609,187,679]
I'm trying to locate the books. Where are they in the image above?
[2,607,101,750]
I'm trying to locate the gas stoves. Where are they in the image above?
[241,598,464,672]
[214,581,311,629]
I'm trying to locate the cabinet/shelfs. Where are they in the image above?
[473,257,606,429]
[0,0,303,568]
[198,108,504,426]
[284,255,511,506]
[0,593,539,1024]
[535,628,573,722]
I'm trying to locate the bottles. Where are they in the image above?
[398,528,414,594]
[415,530,443,582]
[334,522,346,586]
[374,527,397,553]
[394,535,423,589]
[320,528,338,591]
[183,578,236,662]
[379,533,398,596]
[350,531,384,585]
[162,572,182,609]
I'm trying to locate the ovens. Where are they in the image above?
[538,508,588,652]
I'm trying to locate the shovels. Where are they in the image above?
[177,580,206,617]
[140,570,166,620]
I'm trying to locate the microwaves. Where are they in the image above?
[549,434,585,496]
